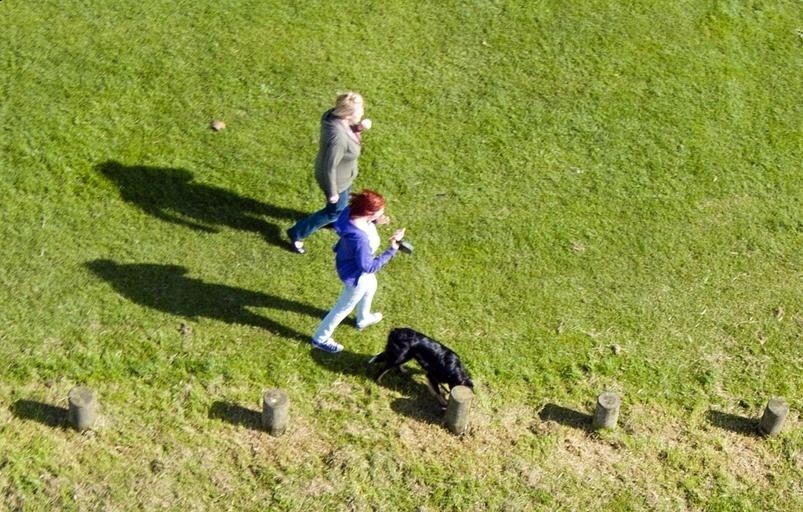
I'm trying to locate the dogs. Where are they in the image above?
[366,327,476,408]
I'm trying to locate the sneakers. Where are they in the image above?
[311,337,345,354]
[355,312,383,332]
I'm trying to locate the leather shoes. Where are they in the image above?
[286,227,307,256]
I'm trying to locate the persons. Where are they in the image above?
[286,90,406,354]
